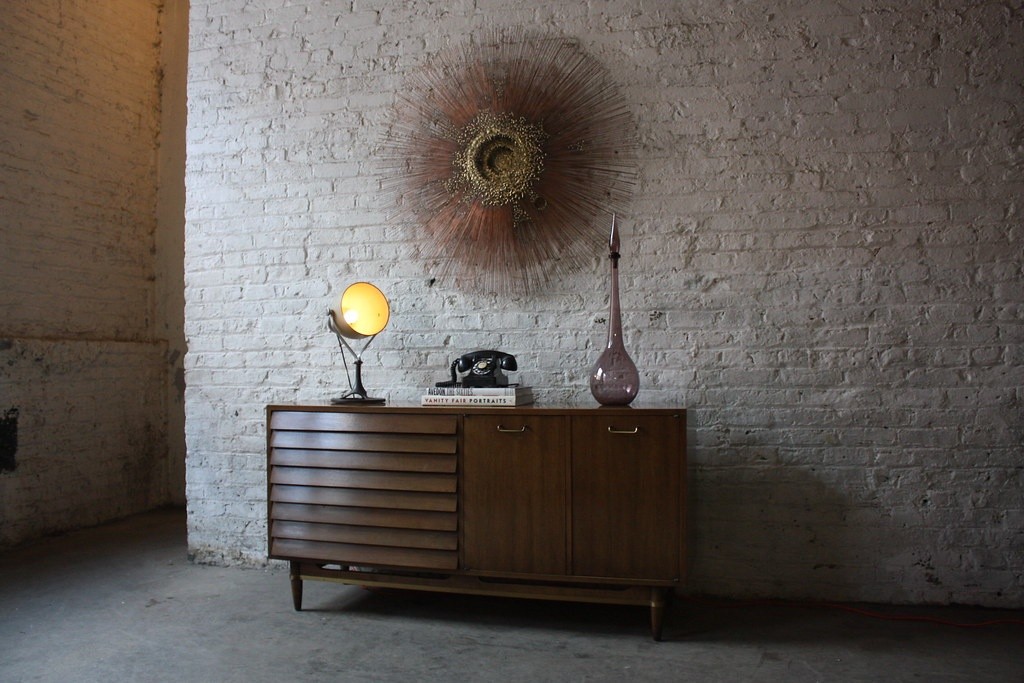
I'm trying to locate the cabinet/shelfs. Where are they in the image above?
[266,404,688,636]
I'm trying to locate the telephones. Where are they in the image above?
[455,350,519,388]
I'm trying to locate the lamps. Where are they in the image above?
[327,282,390,403]
